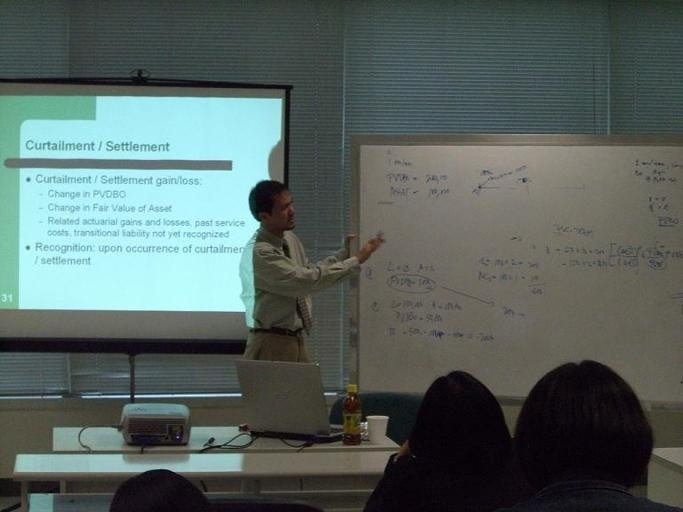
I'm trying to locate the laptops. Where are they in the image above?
[234,359,344,443]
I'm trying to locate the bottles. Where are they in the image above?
[342,384,362,446]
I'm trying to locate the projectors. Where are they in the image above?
[120,403,191,445]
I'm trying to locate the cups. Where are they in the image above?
[366,415,390,445]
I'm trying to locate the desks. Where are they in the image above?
[51,426,400,452]
[11,453,400,512]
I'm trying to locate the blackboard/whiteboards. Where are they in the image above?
[345,133,681,410]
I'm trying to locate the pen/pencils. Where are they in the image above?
[369,240,387,244]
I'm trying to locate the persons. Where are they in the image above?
[236,179,388,363]
[107,468,212,511]
[490,357,682,511]
[363,368,514,512]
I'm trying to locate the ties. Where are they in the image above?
[278,241,314,339]
